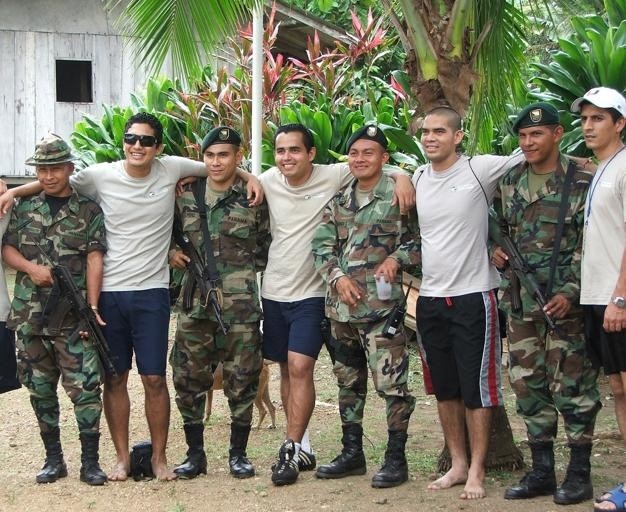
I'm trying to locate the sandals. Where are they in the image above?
[594,488,626,511]
[596,482,624,502]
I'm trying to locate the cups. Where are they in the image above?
[374,273,392,302]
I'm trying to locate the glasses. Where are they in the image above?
[124,133,162,147]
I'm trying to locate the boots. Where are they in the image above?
[315,423,367,479]
[554,447,593,504]
[229,422,254,478]
[36,427,67,483]
[78,432,108,485]
[173,424,207,479]
[372,428,409,488]
[504,441,557,499]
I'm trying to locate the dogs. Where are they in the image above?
[204,359,276,430]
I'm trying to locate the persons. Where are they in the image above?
[0,112,265,483]
[2,136,108,486]
[410,106,599,500]
[168,126,273,479]
[0,178,22,394]
[175,124,416,486]
[312,124,420,488]
[487,102,601,504]
[570,86,626,512]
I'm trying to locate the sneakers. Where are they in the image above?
[270,438,299,486]
[271,448,316,472]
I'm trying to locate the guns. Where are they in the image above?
[45,263,120,379]
[487,206,555,329]
[171,210,231,336]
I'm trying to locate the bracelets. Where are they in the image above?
[90,304,98,310]
[583,156,592,169]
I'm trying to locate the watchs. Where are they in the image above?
[611,295,626,307]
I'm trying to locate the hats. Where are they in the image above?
[346,125,387,154]
[201,127,241,154]
[513,103,560,137]
[23,133,80,166]
[570,87,626,119]
